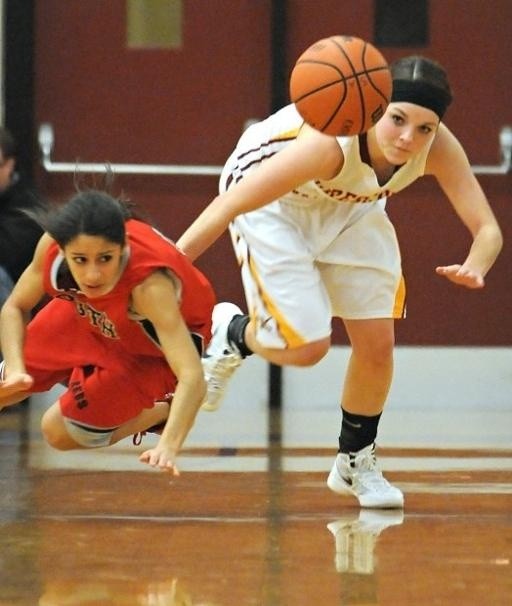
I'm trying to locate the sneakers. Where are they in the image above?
[327,443,404,508]
[145,393,174,435]
[200,302,244,412]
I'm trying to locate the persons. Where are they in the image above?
[1,127,49,323]
[324,508,406,605]
[1,152,219,476]
[175,54,506,510]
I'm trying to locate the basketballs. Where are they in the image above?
[289,34,393,135]
